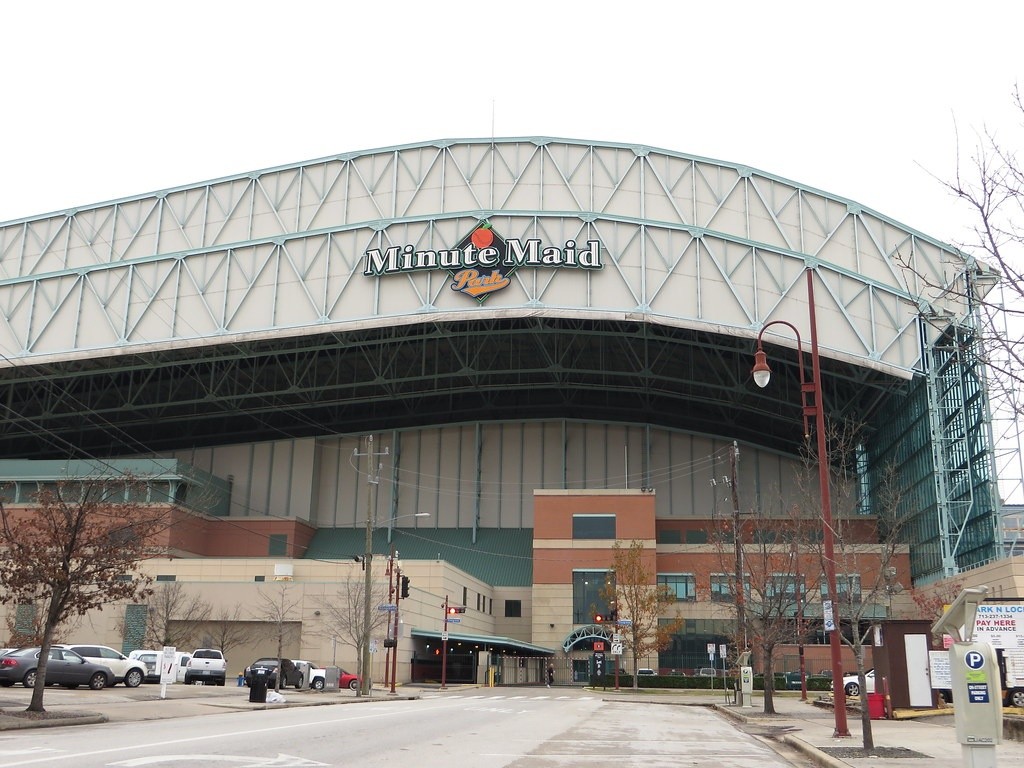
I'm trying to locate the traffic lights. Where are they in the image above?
[448,606,466,614]
[595,615,615,622]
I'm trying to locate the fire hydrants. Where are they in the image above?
[236,672,247,688]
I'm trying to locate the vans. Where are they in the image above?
[128,649,193,684]
[759,670,802,690]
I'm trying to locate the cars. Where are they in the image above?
[0,648,53,660]
[0,646,116,690]
[637,668,657,676]
[699,668,716,678]
[831,670,875,697]
[996,648,1024,708]
[243,658,304,690]
[820,669,832,678]
[320,666,373,691]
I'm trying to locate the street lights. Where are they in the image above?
[750,320,851,741]
[385,559,399,693]
[361,512,431,694]
[441,603,447,690]
[789,532,808,702]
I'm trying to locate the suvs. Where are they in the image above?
[131,679,132,680]
[38,643,150,689]
[184,649,228,687]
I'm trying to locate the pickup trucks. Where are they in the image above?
[291,659,325,692]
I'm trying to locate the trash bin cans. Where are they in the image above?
[868,694,884,720]
[249,668,268,703]
[324,666,341,692]
